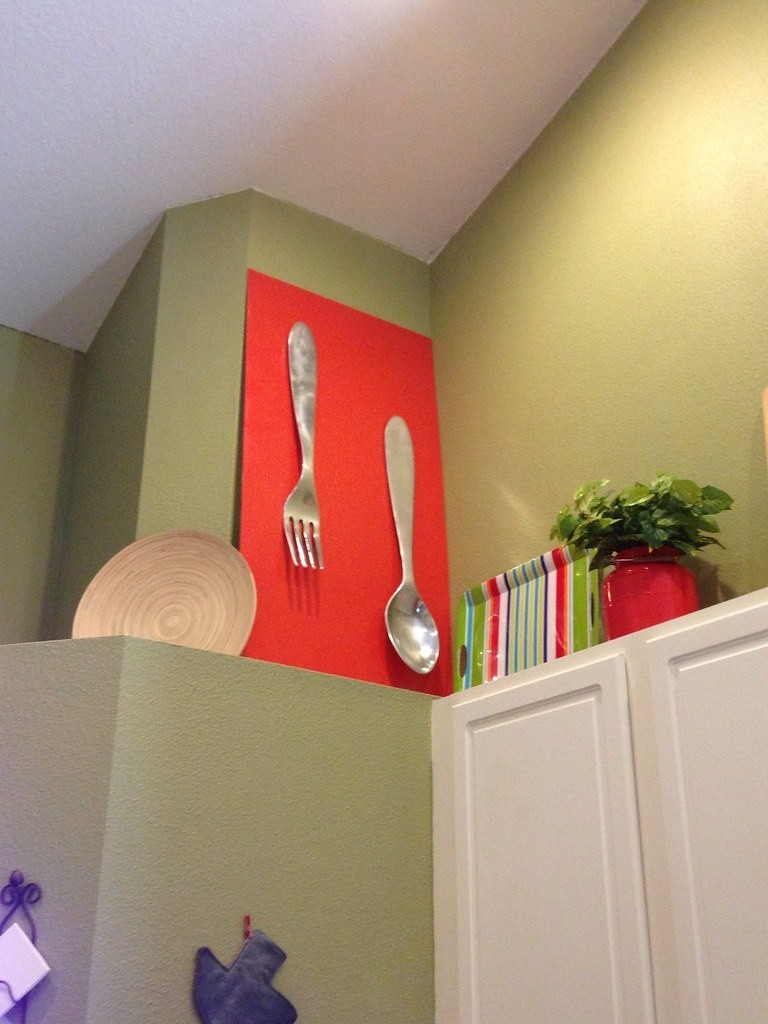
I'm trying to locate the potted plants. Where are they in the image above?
[548,469,738,641]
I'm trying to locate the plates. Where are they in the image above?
[66,533,257,655]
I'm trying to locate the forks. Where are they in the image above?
[280,319,328,570]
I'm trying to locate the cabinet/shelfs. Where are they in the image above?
[430,585,768,1024]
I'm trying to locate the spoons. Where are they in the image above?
[383,414,439,681]
